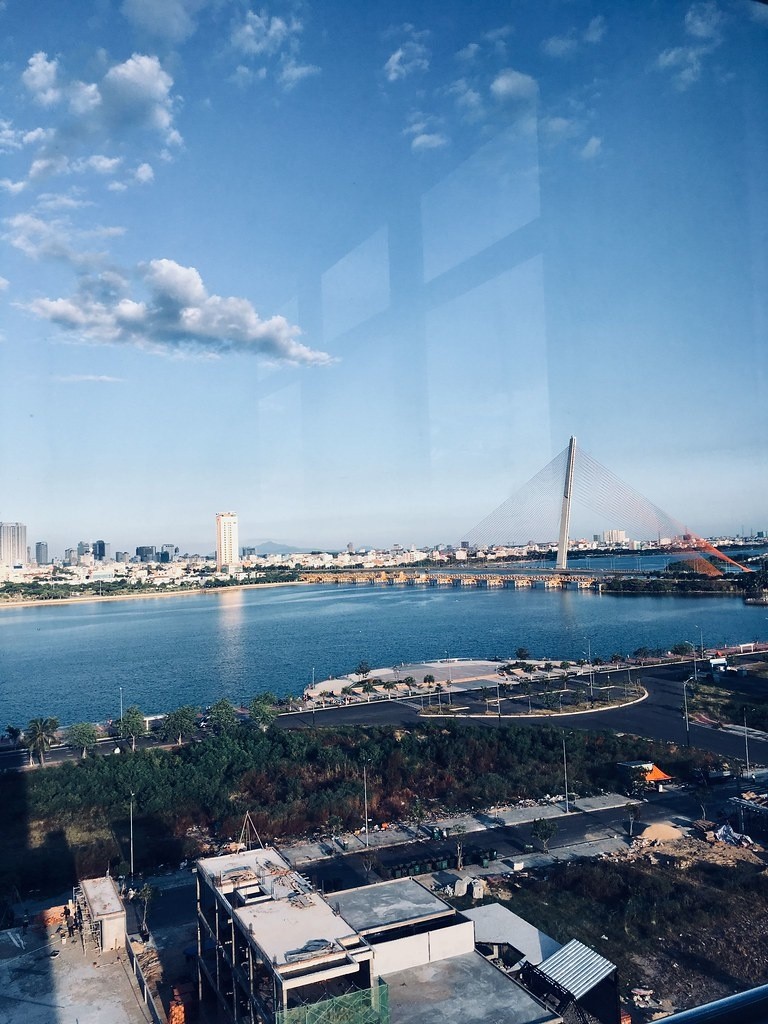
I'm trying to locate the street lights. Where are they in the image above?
[743,707,756,781]
[584,637,591,660]
[684,676,694,733]
[695,625,704,659]
[685,640,698,681]
[120,687,123,739]
[445,651,451,682]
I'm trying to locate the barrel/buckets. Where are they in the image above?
[62,937,66,944]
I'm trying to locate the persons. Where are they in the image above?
[22,914,31,936]
[127,888,135,905]
[61,906,74,937]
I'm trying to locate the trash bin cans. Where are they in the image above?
[343,840,349,851]
[385,846,499,878]
[524,842,536,853]
[434,830,439,841]
